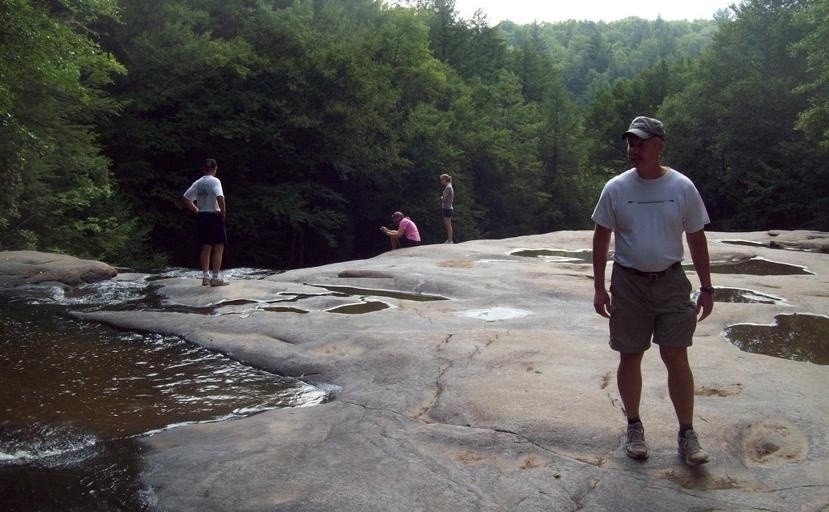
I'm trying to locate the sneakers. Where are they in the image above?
[202,278,229,286]
[677,429,710,467]
[625,422,649,459]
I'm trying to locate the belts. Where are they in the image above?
[622,262,681,281]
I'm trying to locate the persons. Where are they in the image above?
[590,115,715,469]
[439,173,454,244]
[379,207,424,250]
[182,158,231,287]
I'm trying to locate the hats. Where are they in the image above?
[621,116,665,140]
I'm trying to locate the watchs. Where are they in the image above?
[700,286,714,294]
